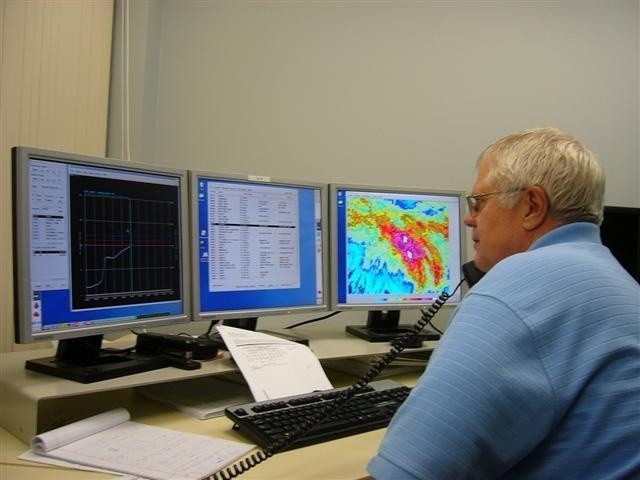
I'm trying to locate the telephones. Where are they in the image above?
[462,260,485,289]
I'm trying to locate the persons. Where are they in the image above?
[367,128,640,479]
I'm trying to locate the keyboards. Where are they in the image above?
[224,378,413,454]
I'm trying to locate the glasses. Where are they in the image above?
[465,185,527,217]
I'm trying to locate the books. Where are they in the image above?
[138,376,257,419]
[17,407,257,480]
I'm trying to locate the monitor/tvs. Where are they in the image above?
[12,146,190,383]
[191,169,330,344]
[331,183,467,343]
